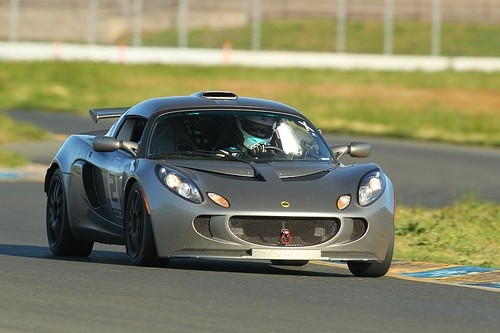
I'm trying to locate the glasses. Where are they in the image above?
[244,122,271,134]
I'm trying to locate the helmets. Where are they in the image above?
[234,115,276,148]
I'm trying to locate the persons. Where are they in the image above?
[209,112,286,161]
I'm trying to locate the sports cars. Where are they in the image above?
[39,89,396,279]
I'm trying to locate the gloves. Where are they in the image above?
[248,141,266,155]
